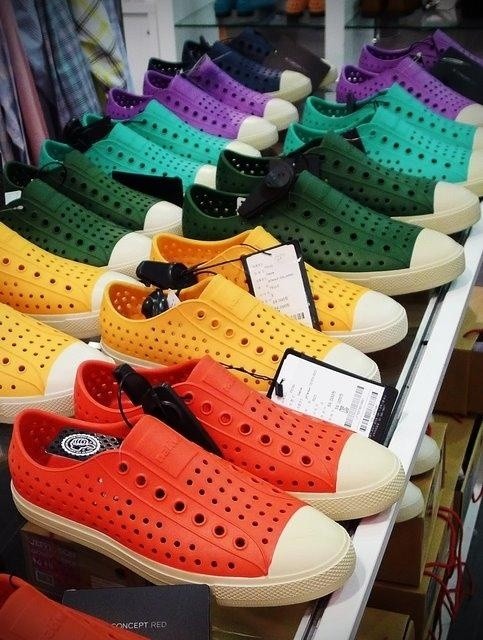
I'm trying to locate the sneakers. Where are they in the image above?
[82,98,262,174]
[148,42,311,103]
[2,302,116,423]
[335,57,482,124]
[105,74,279,151]
[2,224,146,339]
[2,179,154,279]
[302,82,481,152]
[182,26,316,91]
[2,148,184,238]
[216,131,480,235]
[150,226,407,353]
[359,28,481,101]
[143,52,299,130]
[72,356,405,521]
[283,104,481,197]
[38,123,246,218]
[100,275,383,399]
[186,171,464,295]
[8,409,356,604]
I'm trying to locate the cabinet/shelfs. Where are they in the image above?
[18,286,483,640]
[0,188,483,640]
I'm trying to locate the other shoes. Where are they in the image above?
[396,481,424,521]
[410,434,440,475]
